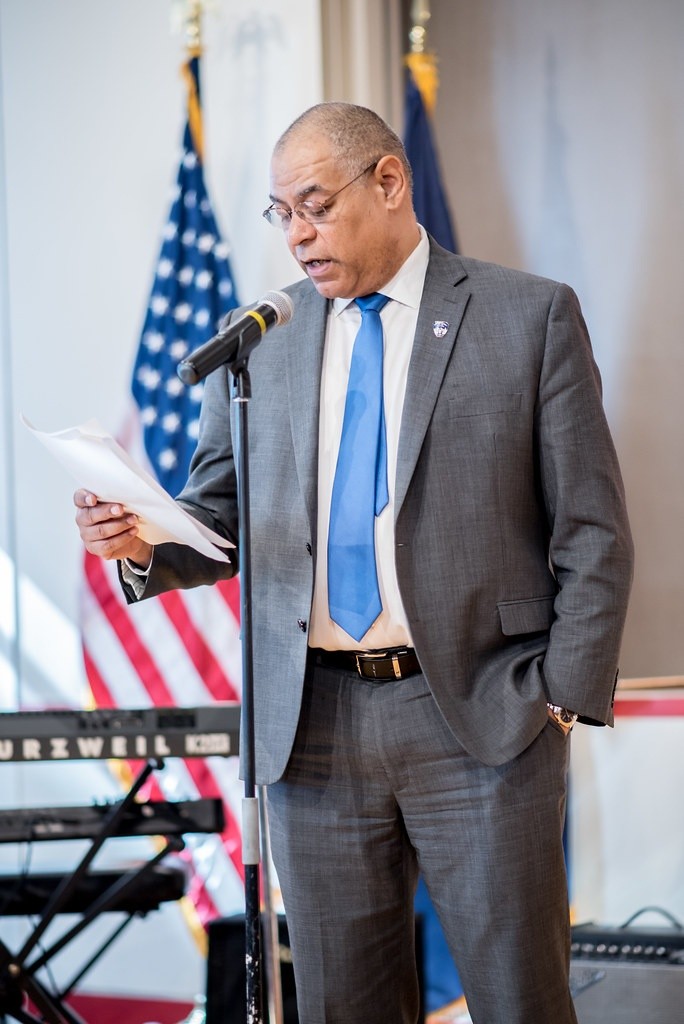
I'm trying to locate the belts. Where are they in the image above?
[306,648,420,681]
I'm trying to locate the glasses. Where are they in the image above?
[262,161,378,230]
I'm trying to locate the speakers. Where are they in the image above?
[568,923,684,1024]
[206,912,428,1024]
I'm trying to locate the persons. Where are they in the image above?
[73,102,634,1023]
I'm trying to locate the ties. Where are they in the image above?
[327,292,390,643]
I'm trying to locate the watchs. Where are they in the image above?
[546,699,580,737]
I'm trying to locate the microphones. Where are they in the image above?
[177,290,294,387]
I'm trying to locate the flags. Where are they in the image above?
[80,0,572,1024]
